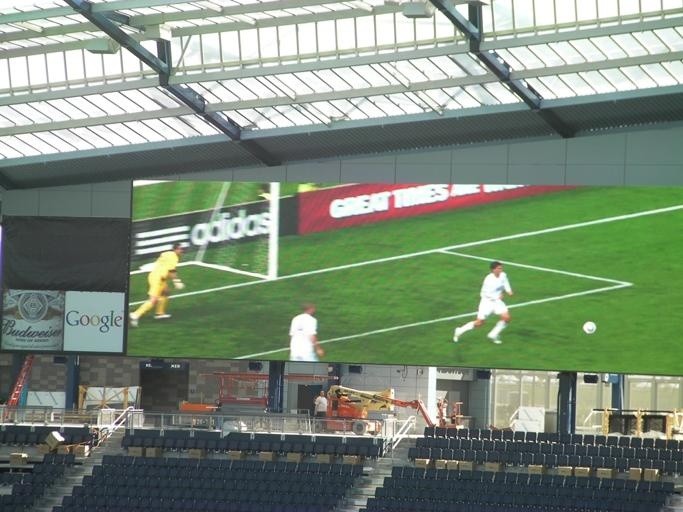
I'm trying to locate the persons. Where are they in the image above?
[128,242,186,328]
[289,302,325,363]
[452,261,513,346]
[314,390,330,432]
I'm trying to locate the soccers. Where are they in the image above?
[583,321,595,334]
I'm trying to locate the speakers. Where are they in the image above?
[583,375,599,384]
[53,356,68,363]
[476,370,490,380]
[151,359,163,366]
[248,362,263,371]
[348,365,362,375]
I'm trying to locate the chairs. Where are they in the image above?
[1,425,683,512]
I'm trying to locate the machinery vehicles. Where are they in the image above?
[313,383,438,434]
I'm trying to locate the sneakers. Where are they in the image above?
[128,311,138,328]
[487,333,503,344]
[452,327,459,342]
[153,314,172,319]
